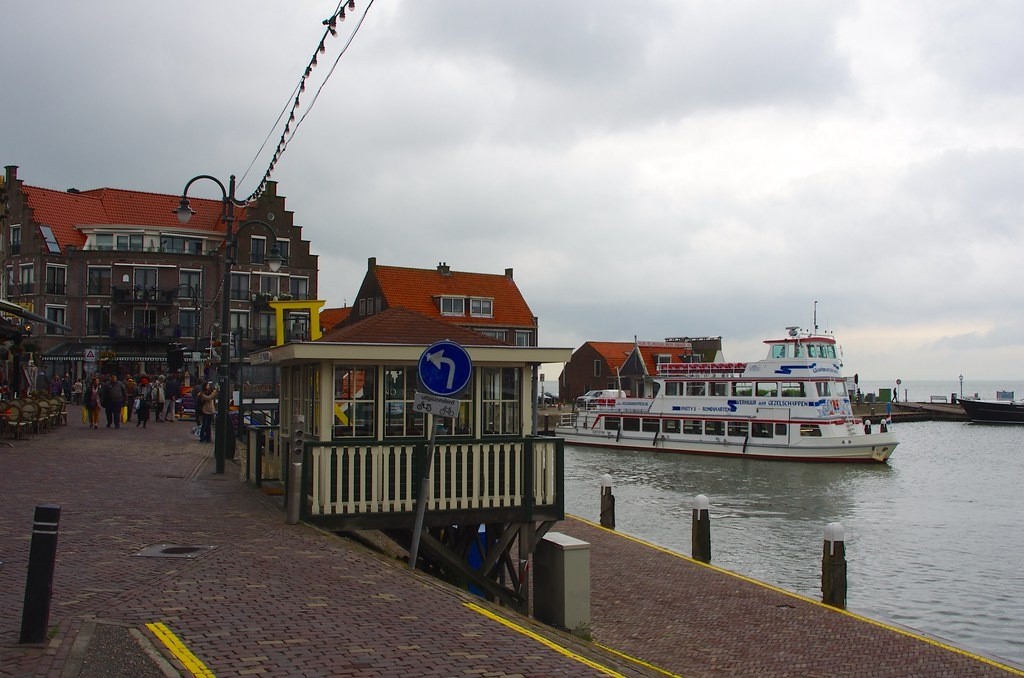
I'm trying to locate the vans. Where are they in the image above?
[576,389,626,407]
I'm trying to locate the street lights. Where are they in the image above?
[7,319,33,399]
[172,175,287,474]
[172,284,223,352]
[959,374,963,398]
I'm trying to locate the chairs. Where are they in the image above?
[0,395,69,440]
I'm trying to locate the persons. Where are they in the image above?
[104,374,127,429]
[191,381,218,444]
[49,373,83,405]
[85,377,103,429]
[121,374,178,429]
[891,388,899,403]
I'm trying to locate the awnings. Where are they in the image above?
[0,299,73,344]
[42,340,222,363]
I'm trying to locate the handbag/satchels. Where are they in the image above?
[152,383,161,404]
[81,405,86,424]
[133,396,141,409]
[121,402,127,424]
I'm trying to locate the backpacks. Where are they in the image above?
[195,393,206,413]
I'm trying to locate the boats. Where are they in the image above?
[553,301,900,464]
[955,399,1024,423]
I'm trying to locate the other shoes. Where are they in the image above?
[90,424,98,429]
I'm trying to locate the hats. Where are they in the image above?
[139,379,149,386]
